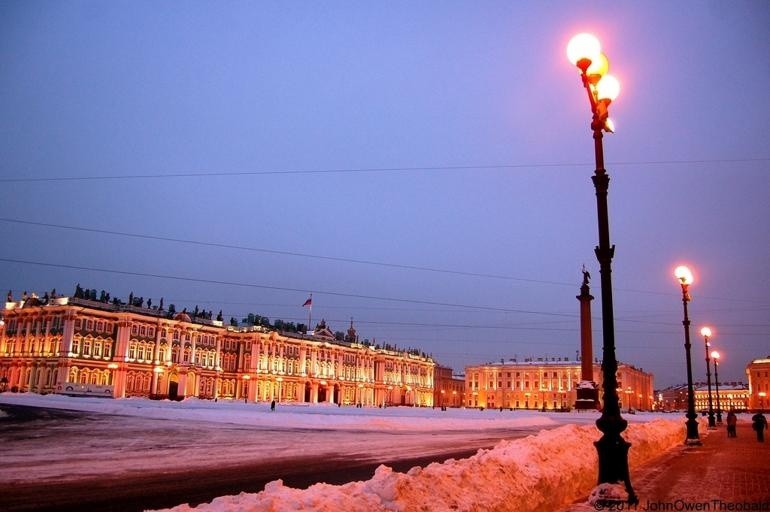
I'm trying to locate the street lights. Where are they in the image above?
[676,266,722,445]
[565,32,639,507]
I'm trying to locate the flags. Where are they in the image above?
[301,297,312,307]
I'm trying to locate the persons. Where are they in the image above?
[751,410,768,443]
[726,411,737,437]
